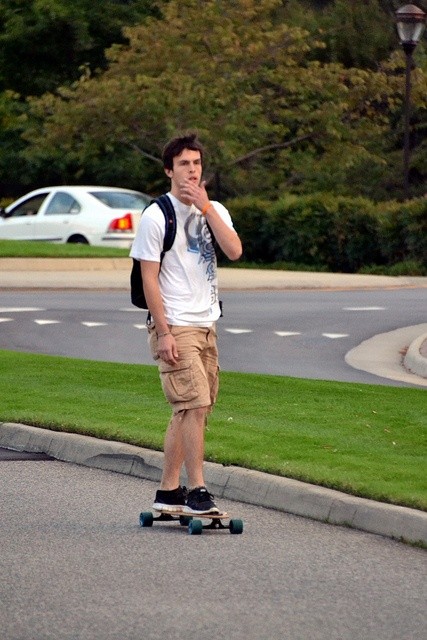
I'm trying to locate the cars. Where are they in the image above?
[0,187,153,249]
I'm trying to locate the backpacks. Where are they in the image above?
[129,195,222,325]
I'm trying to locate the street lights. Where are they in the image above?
[384,2,424,200]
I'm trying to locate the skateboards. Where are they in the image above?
[140,510,243,535]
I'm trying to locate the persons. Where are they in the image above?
[128,135,242,515]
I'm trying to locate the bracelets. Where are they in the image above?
[201,202,212,214]
[159,333,175,336]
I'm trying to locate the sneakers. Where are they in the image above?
[153,484,186,511]
[183,484,219,514]
